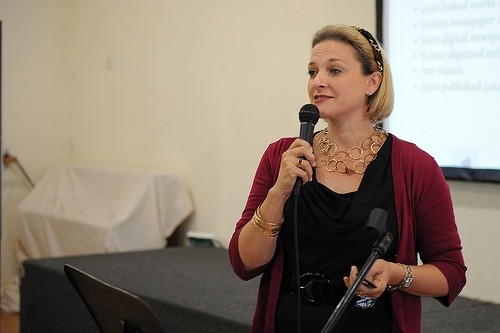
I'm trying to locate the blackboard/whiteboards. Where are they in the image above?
[376,1,500,184]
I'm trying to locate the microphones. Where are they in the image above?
[293,104,320,197]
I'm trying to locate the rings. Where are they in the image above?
[298,158,303,168]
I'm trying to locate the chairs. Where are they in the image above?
[62,264,164,333]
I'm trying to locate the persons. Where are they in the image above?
[229,23,468,333]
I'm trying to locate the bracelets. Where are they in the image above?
[252,203,285,236]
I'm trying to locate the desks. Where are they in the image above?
[19,247,500,333]
[17,164,194,261]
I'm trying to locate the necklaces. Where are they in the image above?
[314,127,387,175]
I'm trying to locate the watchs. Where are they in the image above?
[385,263,414,291]
